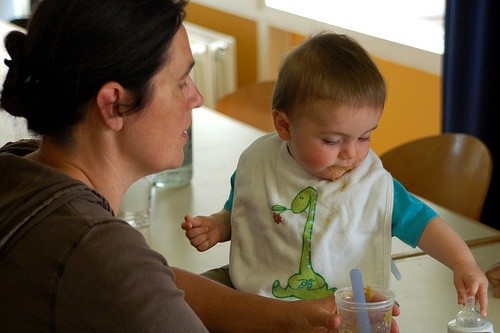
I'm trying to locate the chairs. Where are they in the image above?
[377,132,493,222]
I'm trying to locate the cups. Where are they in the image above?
[333,287,395,333]
[119,175,152,228]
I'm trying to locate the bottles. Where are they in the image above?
[153,114,192,191]
[446,293,494,333]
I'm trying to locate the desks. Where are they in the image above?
[0,16,500,333]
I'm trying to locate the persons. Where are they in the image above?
[0,0,403,333]
[181,32,489,318]
